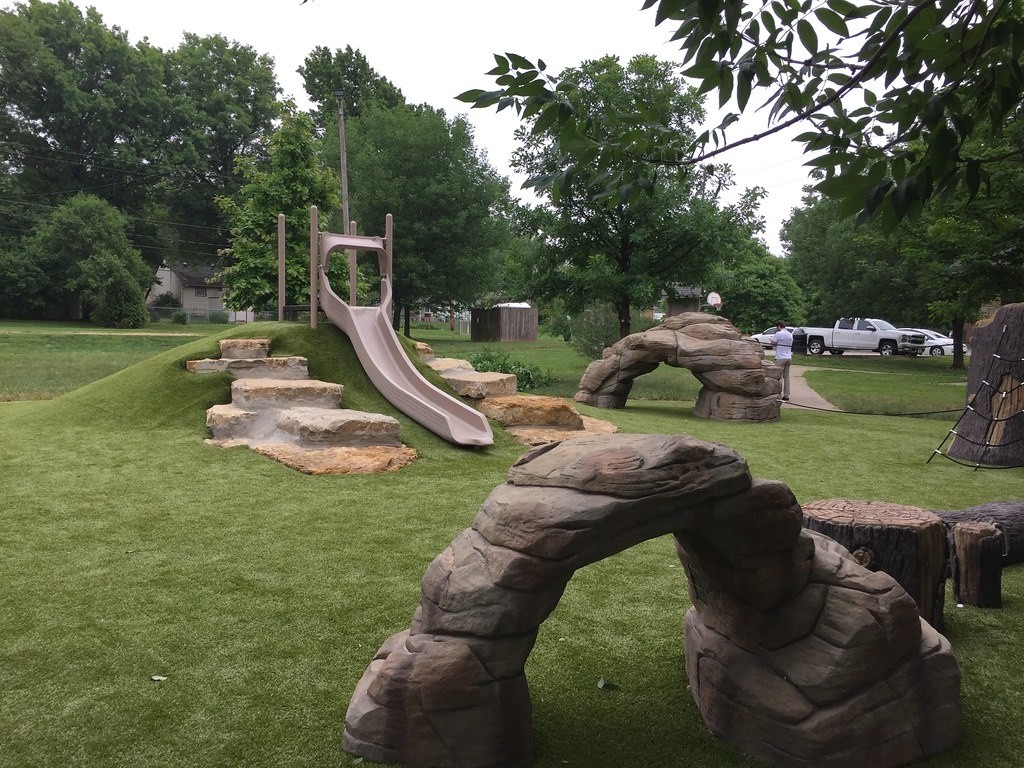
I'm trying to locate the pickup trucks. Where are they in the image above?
[797,317,926,356]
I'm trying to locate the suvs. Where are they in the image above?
[437,307,471,319]
[650,306,666,320]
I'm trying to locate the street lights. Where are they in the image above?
[333,89,351,237]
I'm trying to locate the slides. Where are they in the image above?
[318,275,498,450]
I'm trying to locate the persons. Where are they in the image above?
[771,321,793,400]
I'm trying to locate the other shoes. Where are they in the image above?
[782,397,789,401]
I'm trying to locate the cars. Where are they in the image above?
[750,326,795,350]
[900,328,968,356]
